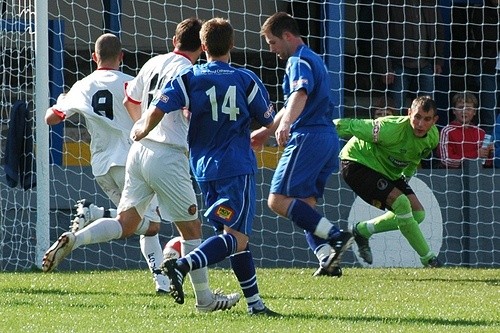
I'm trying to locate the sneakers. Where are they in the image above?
[41,231,76,273]
[324,231,354,270]
[195,287,240,312]
[248,305,283,317]
[420,251,444,268]
[156,286,186,296]
[160,258,185,304]
[351,221,373,264]
[69,198,95,231]
[312,265,343,278]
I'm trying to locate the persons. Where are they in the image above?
[42,17,241,312]
[44,33,171,295]
[357,0,500,169]
[332,95,445,268]
[249,13,353,278]
[131,18,284,317]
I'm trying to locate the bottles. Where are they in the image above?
[478,134,494,165]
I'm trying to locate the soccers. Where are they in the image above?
[163,236,183,259]
